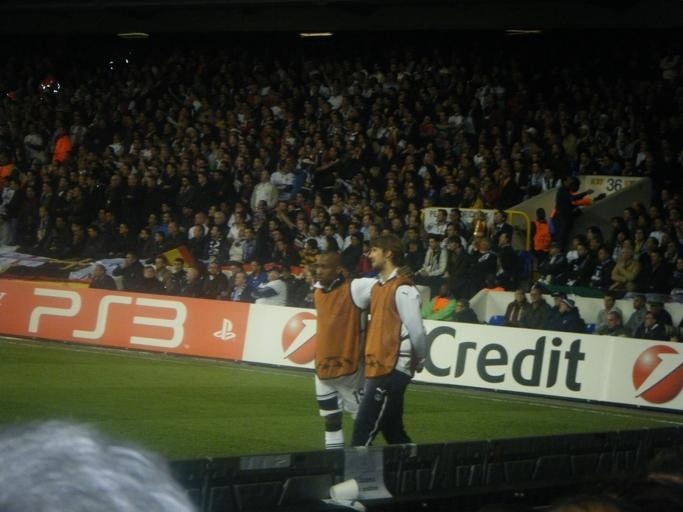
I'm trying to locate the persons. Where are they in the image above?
[0,417,204,512]
[347,234,429,449]
[1,48,683,348]
[308,250,415,453]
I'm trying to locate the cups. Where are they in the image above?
[329,477,359,500]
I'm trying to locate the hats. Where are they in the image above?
[562,298,576,310]
